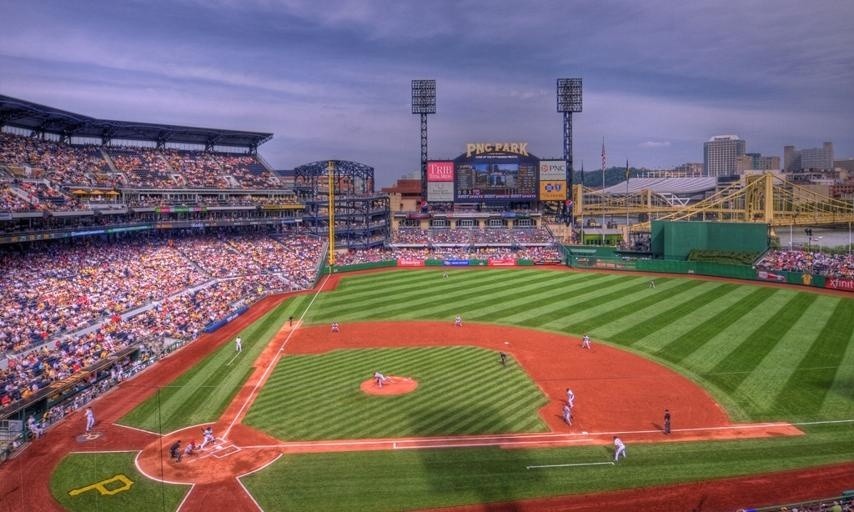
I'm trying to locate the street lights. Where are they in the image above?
[408,79,438,205]
[555,77,583,225]
[805,228,813,253]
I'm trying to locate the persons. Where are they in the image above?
[616,233,652,260]
[757,248,854,279]
[0,132,332,464]
[562,388,574,426]
[582,335,590,349]
[664,409,671,434]
[499,351,506,365]
[737,495,854,512]
[649,280,655,288]
[334,227,561,266]
[372,371,391,388]
[454,314,463,327]
[333,321,339,331]
[613,435,626,461]
